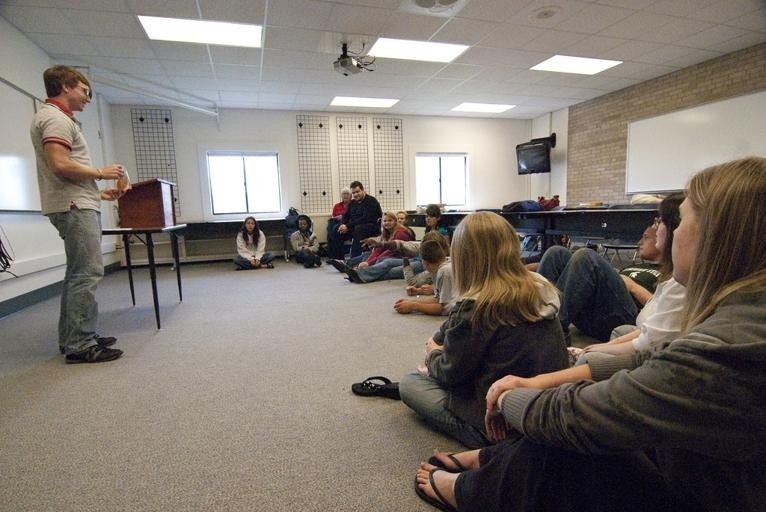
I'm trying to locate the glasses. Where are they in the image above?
[74,85,91,98]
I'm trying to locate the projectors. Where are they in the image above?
[333,40,378,78]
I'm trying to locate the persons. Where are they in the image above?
[326,181,458,316]
[29,64,124,365]
[232,216,275,272]
[413,155,766,512]
[524,186,689,366]
[289,214,323,269]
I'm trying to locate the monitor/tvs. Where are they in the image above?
[516,133,556,175]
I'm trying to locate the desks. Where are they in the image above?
[543,206,660,247]
[101,224,188,329]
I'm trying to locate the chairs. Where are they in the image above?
[520,204,660,269]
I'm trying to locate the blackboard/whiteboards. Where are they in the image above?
[624,86,765,196]
[0,78,83,213]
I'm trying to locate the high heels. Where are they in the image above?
[343,263,357,283]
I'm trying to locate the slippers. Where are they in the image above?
[427,450,468,474]
[413,463,460,511]
[350,375,401,401]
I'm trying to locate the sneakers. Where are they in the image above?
[94,334,118,347]
[64,343,123,365]
